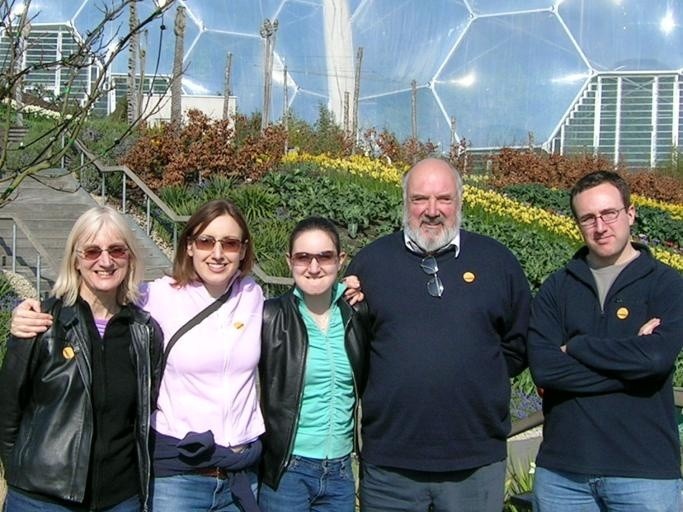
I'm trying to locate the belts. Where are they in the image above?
[187,466,228,480]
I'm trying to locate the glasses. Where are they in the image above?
[188,233,246,253]
[576,205,629,227]
[419,252,444,298]
[76,243,130,260]
[289,250,340,266]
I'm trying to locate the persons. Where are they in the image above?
[341,156,533,512]
[9,195,366,512]
[257,215,371,512]
[525,169,683,512]
[0,205,165,512]
